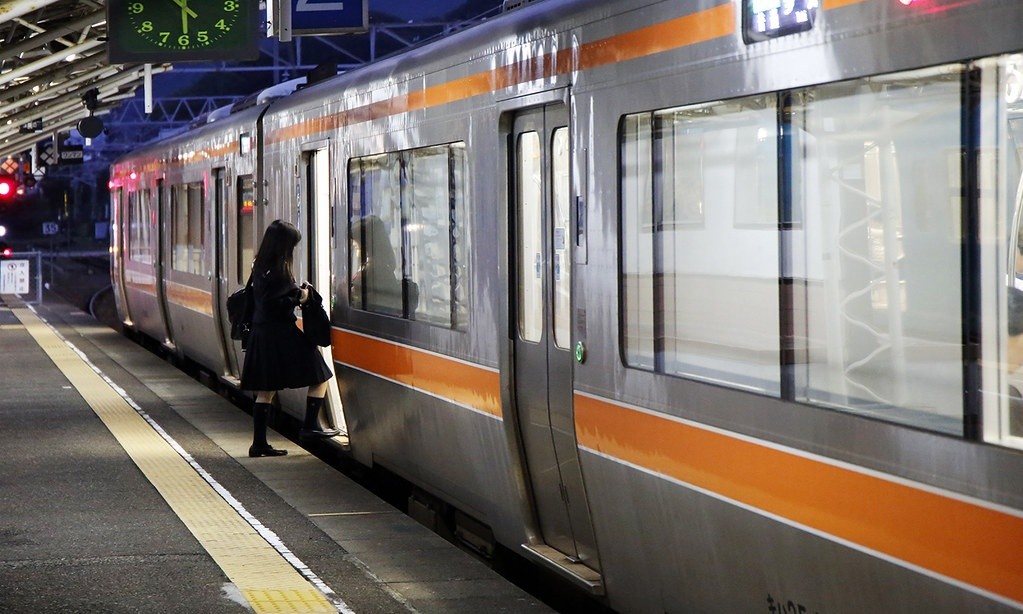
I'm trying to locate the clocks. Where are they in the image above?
[104,0,260,64]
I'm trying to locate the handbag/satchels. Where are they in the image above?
[299,280,331,347]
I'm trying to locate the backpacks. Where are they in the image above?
[226,265,254,341]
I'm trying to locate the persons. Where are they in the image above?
[1007,227,1023,437]
[350,214,402,315]
[239,220,340,457]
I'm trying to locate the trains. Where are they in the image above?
[104,0,1023,614]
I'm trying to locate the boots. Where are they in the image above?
[304,396,340,437]
[249,403,288,457]
